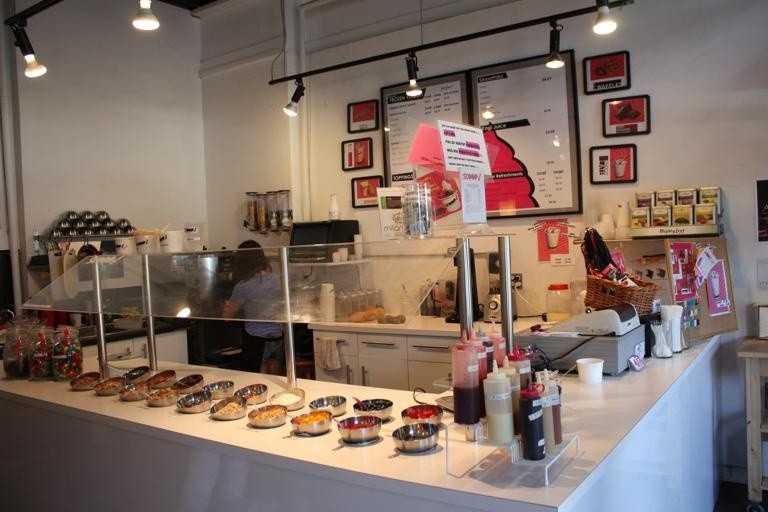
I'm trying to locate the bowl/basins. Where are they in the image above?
[68,365,445,455]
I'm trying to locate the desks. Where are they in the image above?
[735,336,768,511]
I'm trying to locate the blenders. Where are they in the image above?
[483,253,517,324]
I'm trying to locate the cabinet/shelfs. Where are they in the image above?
[407,334,462,394]
[312,329,409,392]
[82,328,189,373]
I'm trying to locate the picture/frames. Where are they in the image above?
[601,94,651,138]
[347,98,380,134]
[754,177,768,244]
[351,175,384,208]
[588,143,638,184]
[340,137,373,171]
[583,49,632,96]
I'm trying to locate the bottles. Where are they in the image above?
[400,284,411,315]
[328,192,341,222]
[546,284,572,323]
[3,314,83,382]
[451,321,563,461]
[420,282,442,316]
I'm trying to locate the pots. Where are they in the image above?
[76,241,99,282]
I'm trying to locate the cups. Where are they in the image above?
[332,233,362,262]
[585,200,632,241]
[355,143,365,165]
[289,277,383,320]
[111,219,210,257]
[544,227,560,249]
[438,189,461,212]
[709,274,720,297]
[613,159,626,179]
[360,182,369,197]
[650,307,690,359]
[68,312,89,327]
[399,179,433,237]
[575,357,606,385]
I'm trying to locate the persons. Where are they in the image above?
[221,239,287,377]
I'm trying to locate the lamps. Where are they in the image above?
[268,0,636,117]
[3,0,160,79]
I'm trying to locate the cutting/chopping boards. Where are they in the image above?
[46,247,79,302]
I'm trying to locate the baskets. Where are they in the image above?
[584,274,662,316]
